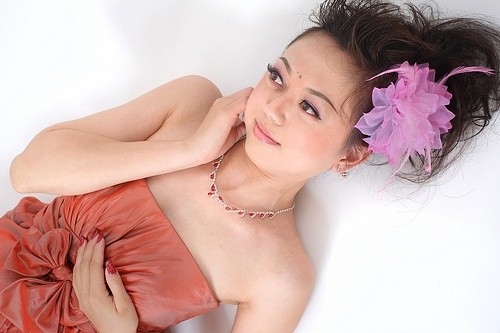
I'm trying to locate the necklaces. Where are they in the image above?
[207,156,296,220]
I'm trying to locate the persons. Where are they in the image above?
[0,1,500,333]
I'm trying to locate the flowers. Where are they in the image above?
[354,60,496,187]
